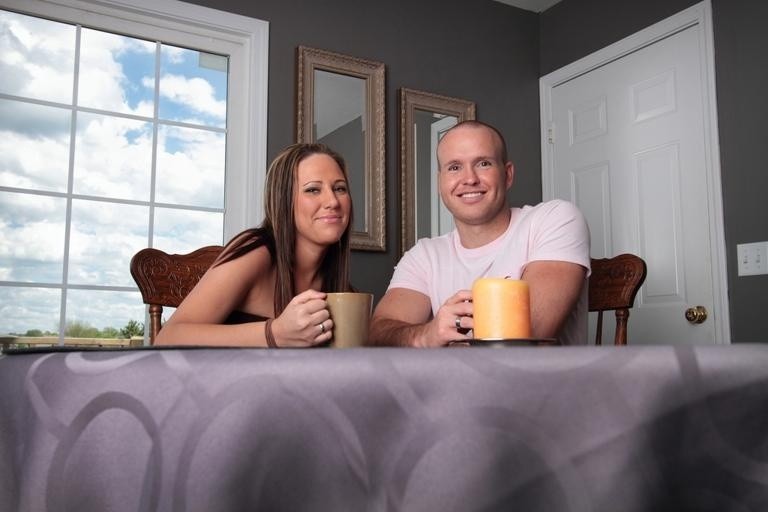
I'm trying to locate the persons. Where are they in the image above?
[364,119,592,348]
[154,141,357,348]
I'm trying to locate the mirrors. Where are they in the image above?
[398,87,476,253]
[294,46,389,250]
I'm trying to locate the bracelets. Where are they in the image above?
[264,318,277,349]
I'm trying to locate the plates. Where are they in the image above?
[452,336,558,347]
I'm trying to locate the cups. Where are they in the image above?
[324,292,374,347]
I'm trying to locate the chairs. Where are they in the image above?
[586,253,647,347]
[129,246,223,344]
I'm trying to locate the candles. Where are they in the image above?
[473,276,531,340]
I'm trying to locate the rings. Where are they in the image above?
[319,323,325,333]
[455,317,461,328]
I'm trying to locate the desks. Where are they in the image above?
[1,342,768,510]
